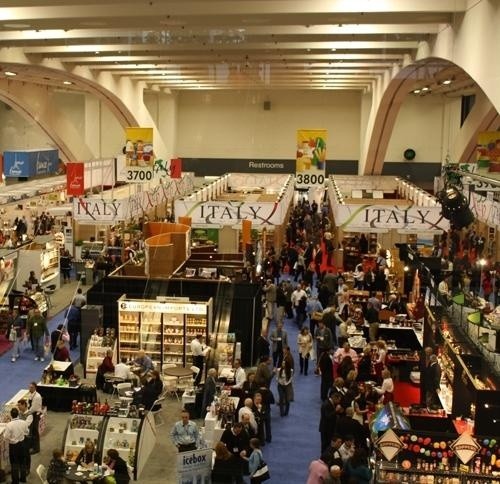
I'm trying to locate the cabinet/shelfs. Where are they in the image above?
[17,248,60,293]
[62,413,143,480]
[434,324,482,415]
[117,294,214,370]
[9,291,48,319]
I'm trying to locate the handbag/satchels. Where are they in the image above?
[248,451,270,484]
[9,329,17,341]
[309,349,317,361]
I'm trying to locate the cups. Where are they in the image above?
[94,463,98,473]
[99,466,103,474]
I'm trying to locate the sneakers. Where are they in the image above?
[40,357,44,361]
[11,357,15,362]
[34,357,39,360]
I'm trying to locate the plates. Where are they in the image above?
[77,465,87,472]
[75,473,83,476]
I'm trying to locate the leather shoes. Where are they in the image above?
[30,450,40,455]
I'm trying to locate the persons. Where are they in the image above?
[170,412,199,452]
[211,198,499,483]
[4,409,27,484]
[47,442,129,483]
[27,383,42,454]
[16,401,34,475]
[190,334,210,386]
[1,209,164,412]
[200,368,218,418]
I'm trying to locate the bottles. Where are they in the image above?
[375,455,500,484]
[210,395,235,428]
[110,399,146,420]
[70,399,109,415]
